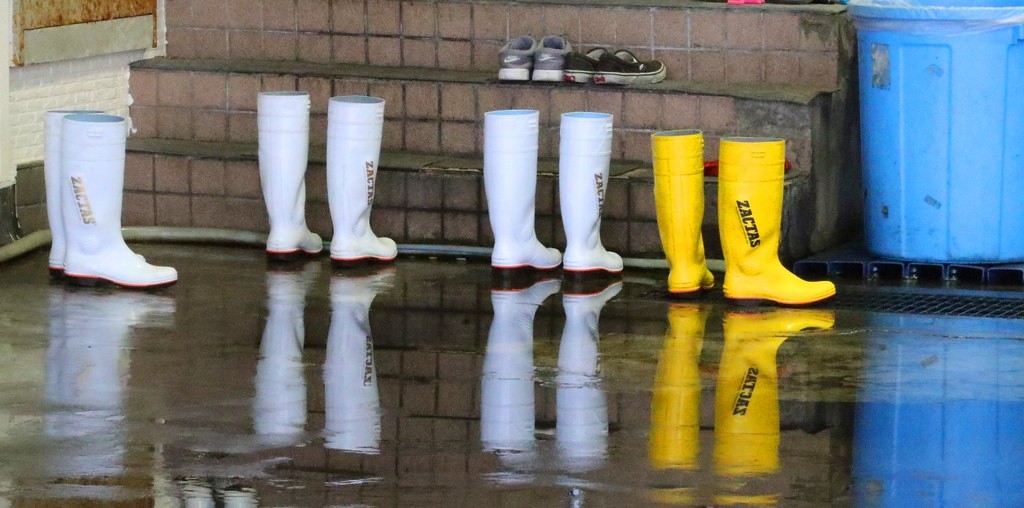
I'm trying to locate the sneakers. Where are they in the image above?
[564,47,608,84]
[532,35,572,82]
[497,36,537,81]
[594,50,666,84]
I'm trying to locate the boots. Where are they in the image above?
[326,96,398,266]
[480,280,559,453]
[650,129,714,298]
[61,114,178,290]
[483,109,562,277]
[554,280,630,467]
[256,91,323,260]
[253,263,318,450]
[41,285,179,495]
[559,112,625,278]
[648,304,716,505]
[718,136,836,307]
[43,109,104,272]
[712,308,836,505]
[323,269,400,456]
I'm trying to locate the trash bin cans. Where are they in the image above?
[847,1,1024,265]
